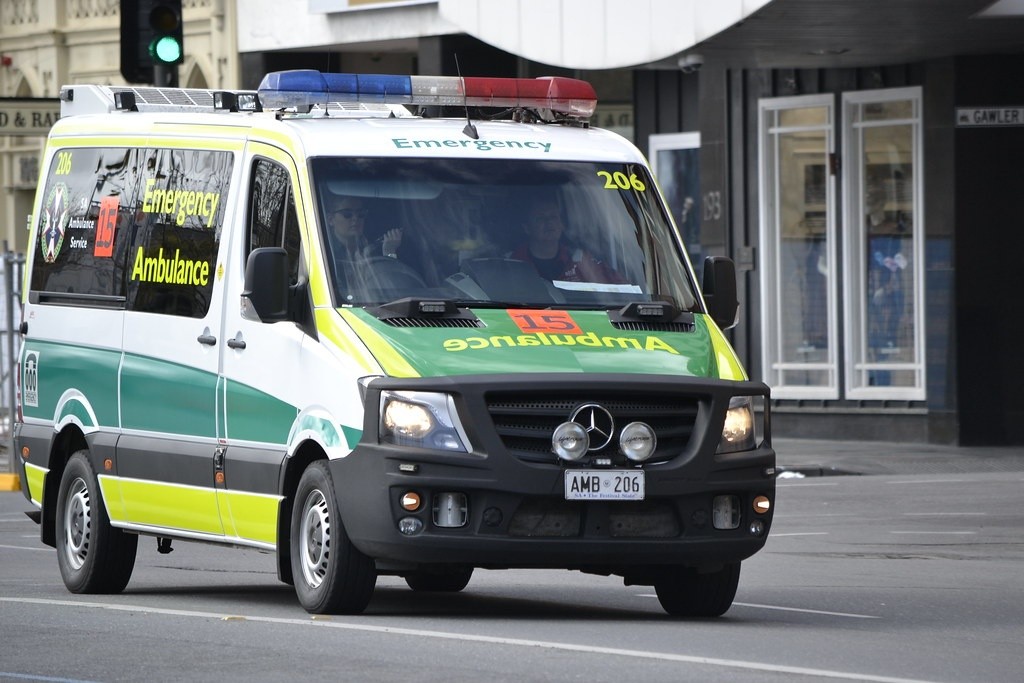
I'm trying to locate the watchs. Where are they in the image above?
[387,253,398,259]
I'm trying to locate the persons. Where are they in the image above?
[325,194,406,303]
[501,190,632,308]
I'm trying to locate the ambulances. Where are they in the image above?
[15,67,780,617]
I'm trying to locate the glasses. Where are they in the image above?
[333,207,369,219]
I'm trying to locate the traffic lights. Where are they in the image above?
[139,0,185,66]
